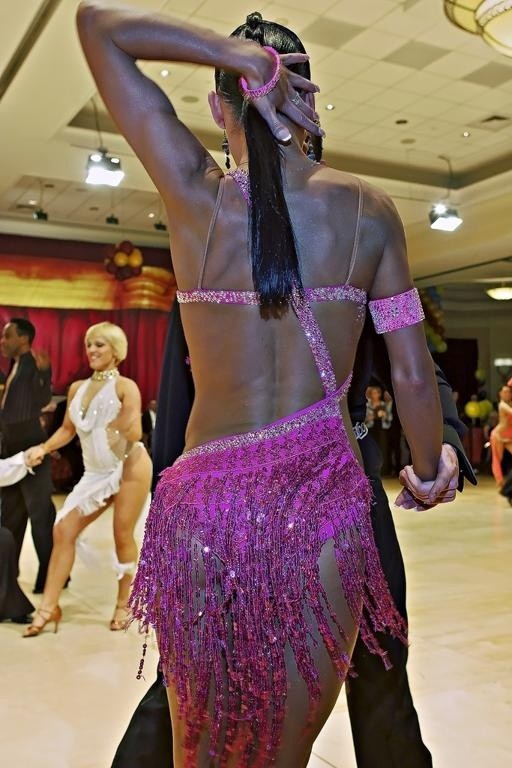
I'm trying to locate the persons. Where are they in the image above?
[108,118,477,768]
[366,367,512,507]
[76,2,442,767]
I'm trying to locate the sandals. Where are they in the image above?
[110,602,136,631]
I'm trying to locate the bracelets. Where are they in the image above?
[237,45,283,103]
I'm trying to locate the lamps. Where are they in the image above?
[441,0,512,57]
[82,96,125,190]
[428,154,465,233]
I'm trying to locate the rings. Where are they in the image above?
[293,91,304,106]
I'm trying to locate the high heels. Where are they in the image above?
[21,607,62,638]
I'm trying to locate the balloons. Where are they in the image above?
[105,238,143,282]
[418,287,451,359]
